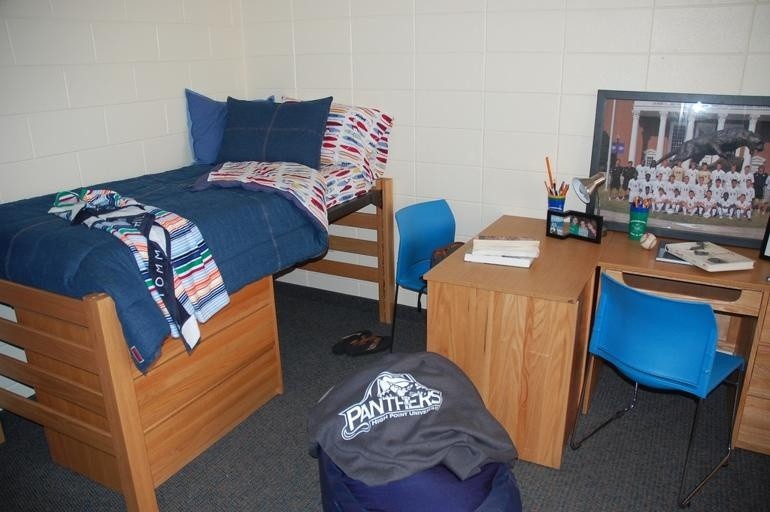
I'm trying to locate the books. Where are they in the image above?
[656,241,693,266]
[663,242,756,274]
[463,235,540,269]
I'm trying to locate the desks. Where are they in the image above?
[423,216,770,470]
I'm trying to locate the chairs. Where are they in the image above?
[389,199,457,353]
[568,273,746,507]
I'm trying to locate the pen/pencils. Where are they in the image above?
[544,155,569,196]
[633,196,650,209]
[695,250,730,255]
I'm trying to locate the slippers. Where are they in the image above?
[331,329,372,355]
[345,335,392,356]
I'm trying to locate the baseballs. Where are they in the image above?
[640,232,656,249]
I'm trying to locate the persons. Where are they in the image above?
[586,222,597,240]
[578,219,589,238]
[609,158,769,223]
[569,216,580,235]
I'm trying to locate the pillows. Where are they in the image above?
[185,88,394,180]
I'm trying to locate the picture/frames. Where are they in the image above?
[758,215,770,261]
[585,89,770,249]
[546,209,603,244]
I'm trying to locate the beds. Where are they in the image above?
[0,162,396,512]
